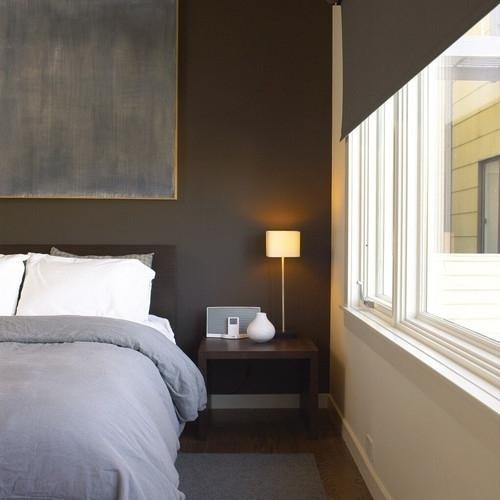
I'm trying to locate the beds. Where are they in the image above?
[0,243,208,500]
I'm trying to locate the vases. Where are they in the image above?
[247,312,276,344]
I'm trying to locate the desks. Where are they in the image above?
[197,338,319,440]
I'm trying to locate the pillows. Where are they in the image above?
[0,254,26,316]
[49,246,154,268]
[15,252,156,323]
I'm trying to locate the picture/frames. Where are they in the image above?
[0,0,179,201]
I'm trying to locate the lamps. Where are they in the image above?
[266,230,301,339]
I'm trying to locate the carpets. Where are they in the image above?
[174,453,327,500]
